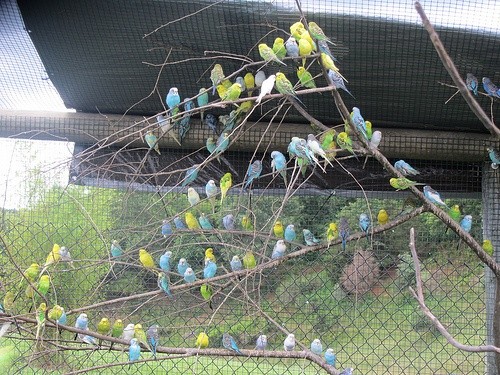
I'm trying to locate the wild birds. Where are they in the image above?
[110,239,123,269]
[486,140,500,165]
[482,239,494,258]
[466,72,500,99]
[3,243,72,339]
[193,331,356,375]
[137,21,424,313]
[73,312,160,371]
[423,186,473,234]
